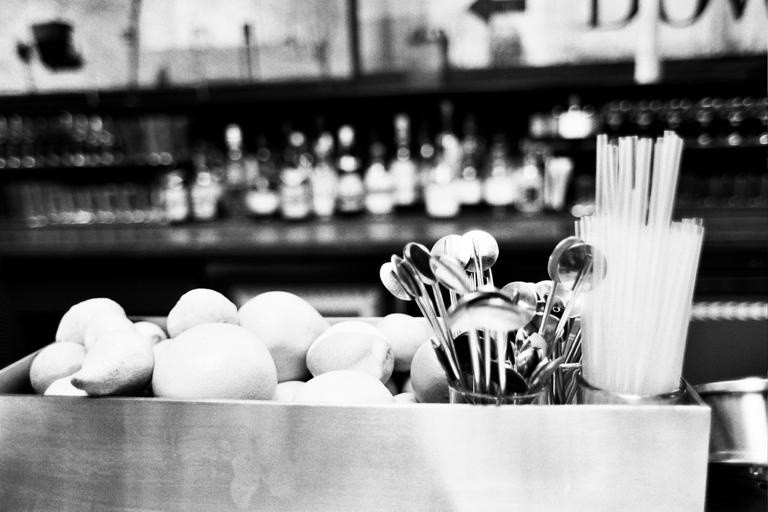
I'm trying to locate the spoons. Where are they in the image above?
[376,223,605,393]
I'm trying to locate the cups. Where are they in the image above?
[575,378,687,405]
[447,385,550,405]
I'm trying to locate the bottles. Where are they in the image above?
[1,96,767,228]
[402,1,450,90]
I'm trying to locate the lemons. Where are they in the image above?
[29,288,452,404]
